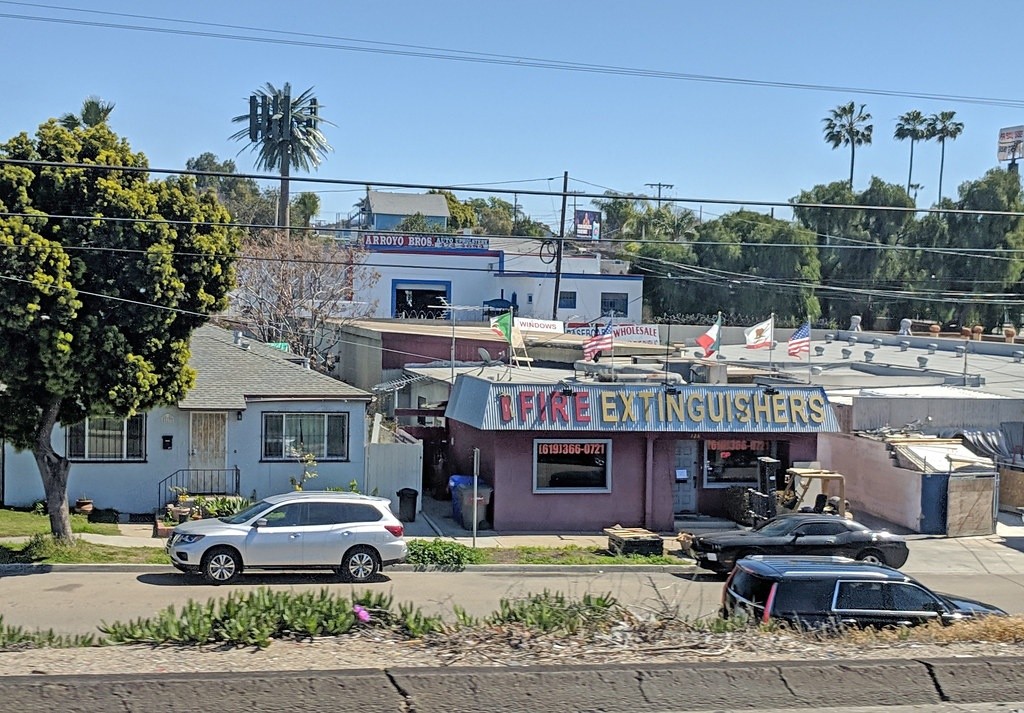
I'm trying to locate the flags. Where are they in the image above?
[788,320,811,359]
[744,317,774,349]
[695,316,720,358]
[490,312,512,344]
[582,318,614,362]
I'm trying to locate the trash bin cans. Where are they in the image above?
[450,475,492,531]
[397,488,419,523]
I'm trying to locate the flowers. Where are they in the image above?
[177,495,187,509]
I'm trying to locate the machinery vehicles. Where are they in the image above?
[743,456,853,529]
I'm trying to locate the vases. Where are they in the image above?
[171,507,190,520]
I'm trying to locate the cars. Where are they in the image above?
[689,513,909,575]
[548,468,606,489]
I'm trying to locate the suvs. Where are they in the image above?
[717,553,1011,633]
[167,490,409,583]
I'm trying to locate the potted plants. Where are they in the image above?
[192,495,205,520]
[179,507,189,524]
[76,491,93,509]
[167,485,189,502]
